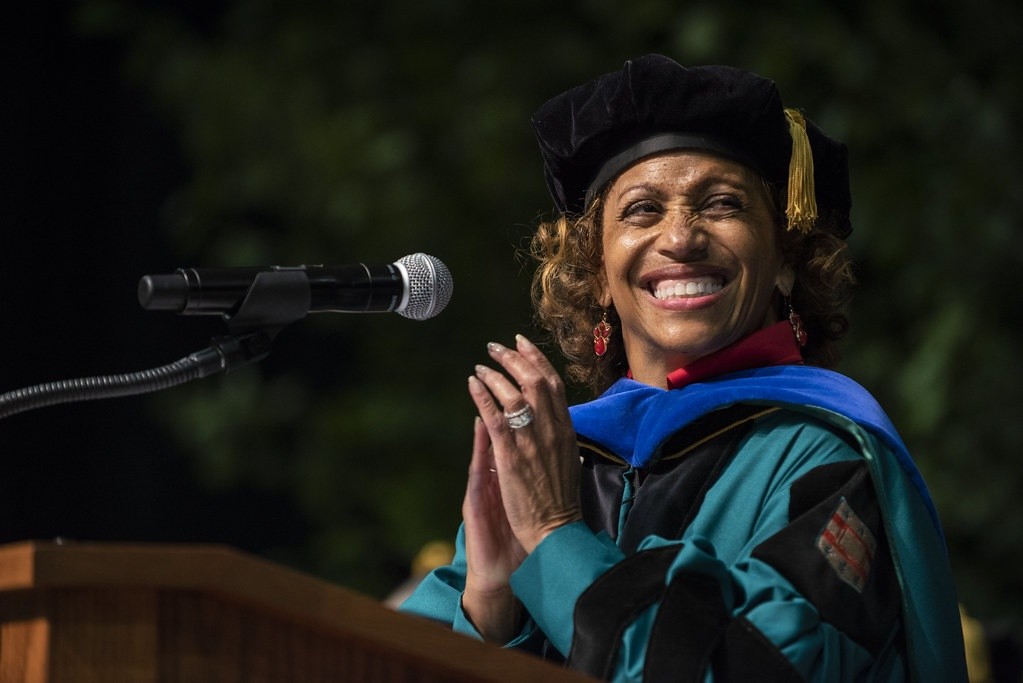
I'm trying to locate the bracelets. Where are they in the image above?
[488,468,497,473]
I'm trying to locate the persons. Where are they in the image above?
[396,53,970,683]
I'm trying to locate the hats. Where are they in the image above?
[531,54,855,247]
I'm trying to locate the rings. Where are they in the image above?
[505,404,534,429]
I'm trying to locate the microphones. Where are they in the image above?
[137,252,453,321]
